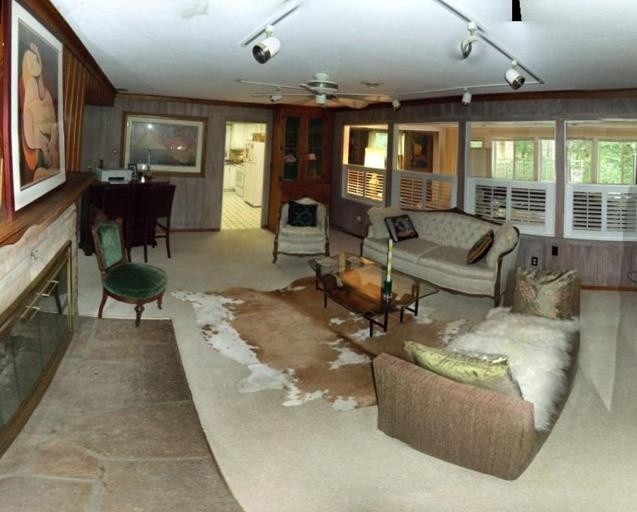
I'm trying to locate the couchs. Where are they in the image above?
[357,205,521,309]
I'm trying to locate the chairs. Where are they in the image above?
[271,198,334,264]
[91,183,177,329]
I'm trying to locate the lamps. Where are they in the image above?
[390,92,403,113]
[269,85,283,103]
[250,24,281,66]
[452,22,526,109]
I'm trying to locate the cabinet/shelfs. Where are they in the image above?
[266,105,334,235]
[78,186,155,257]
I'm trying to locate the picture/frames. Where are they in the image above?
[117,111,209,179]
[0,0,68,226]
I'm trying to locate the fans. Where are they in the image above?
[236,72,383,106]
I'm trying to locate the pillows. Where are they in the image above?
[287,202,318,228]
[397,340,523,399]
[465,221,518,272]
[510,266,579,322]
[366,205,419,243]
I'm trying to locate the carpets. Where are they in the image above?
[171,274,465,411]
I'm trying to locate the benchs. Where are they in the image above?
[372,266,583,484]
